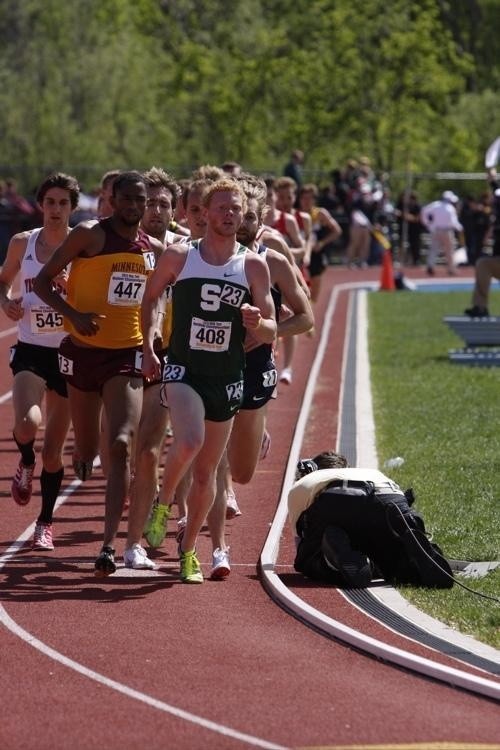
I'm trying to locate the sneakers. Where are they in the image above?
[32,521,54,550]
[12,461,36,506]
[74,434,269,584]
[280,369,291,385]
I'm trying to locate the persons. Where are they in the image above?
[287,452,454,589]
[343,136,500,319]
[206,180,315,578]
[95,150,343,542]
[32,171,169,573]
[0,173,80,550]
[140,179,278,584]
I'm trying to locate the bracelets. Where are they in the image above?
[247,318,261,330]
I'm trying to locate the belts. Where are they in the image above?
[327,480,398,489]
